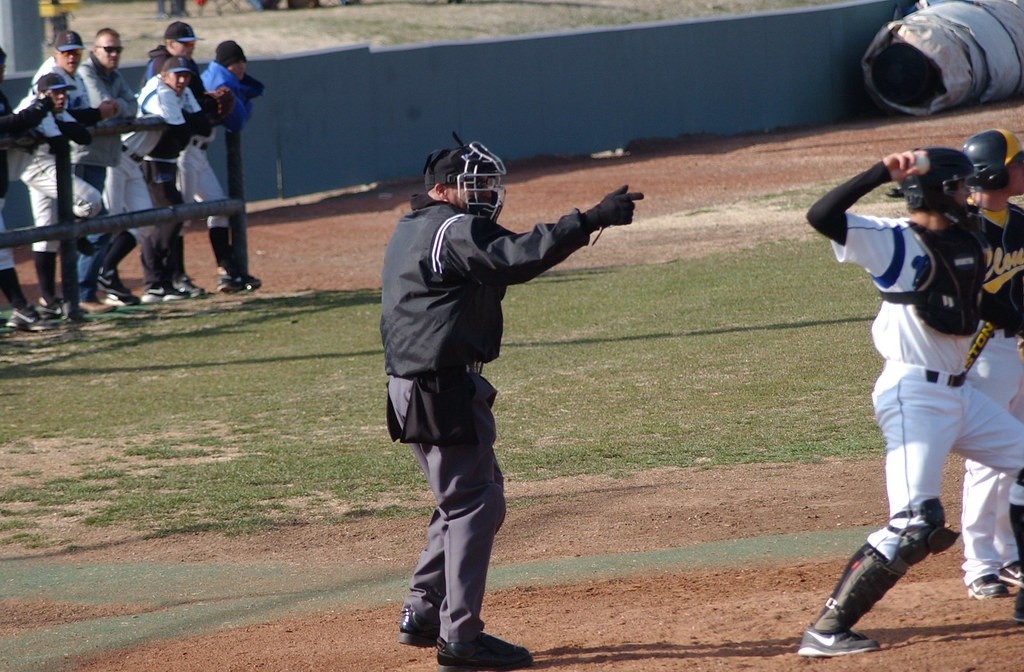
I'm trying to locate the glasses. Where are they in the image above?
[93,45,123,53]
[61,49,81,56]
[52,89,66,96]
[181,41,195,48]
[465,176,497,190]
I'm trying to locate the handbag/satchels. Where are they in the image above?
[400,372,480,446]
[385,379,402,443]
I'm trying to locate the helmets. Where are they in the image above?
[959,128,1024,185]
[900,146,982,232]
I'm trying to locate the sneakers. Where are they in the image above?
[398,607,484,647]
[436,635,532,672]
[998,563,1024,588]
[1013,608,1024,622]
[0,268,262,332]
[798,627,881,657]
[967,574,1008,600]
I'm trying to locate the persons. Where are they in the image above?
[379,141,645,672]
[5,73,92,182]
[961,128,1024,598]
[28,30,118,315]
[96,55,211,306]
[141,21,235,303]
[171,39,265,297]
[78,27,138,314]
[798,148,1024,655]
[0,46,56,332]
[155,0,209,19]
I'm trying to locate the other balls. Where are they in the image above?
[908,154,929,174]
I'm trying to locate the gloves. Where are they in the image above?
[582,184,644,231]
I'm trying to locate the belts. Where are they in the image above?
[121,145,144,163]
[406,360,484,376]
[926,369,965,386]
[192,140,208,151]
[989,326,1024,338]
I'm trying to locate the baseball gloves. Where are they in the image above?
[204,85,235,120]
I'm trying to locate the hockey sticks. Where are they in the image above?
[884,323,995,387]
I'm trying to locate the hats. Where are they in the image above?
[423,145,498,187]
[165,22,197,42]
[55,31,85,51]
[38,73,76,91]
[162,57,194,72]
[215,40,247,68]
